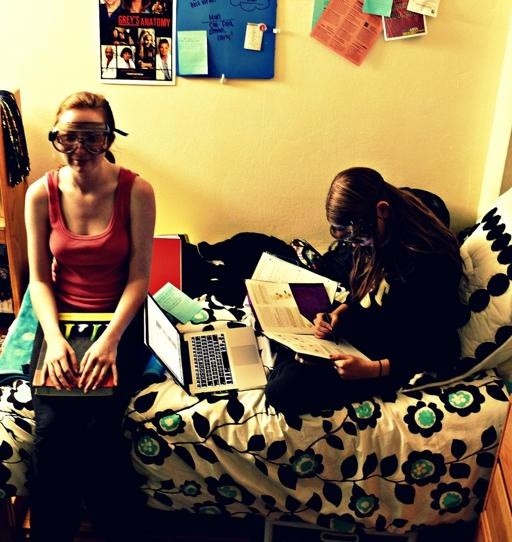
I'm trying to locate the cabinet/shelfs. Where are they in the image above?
[0,88,31,348]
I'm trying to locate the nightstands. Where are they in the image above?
[472,395,511,542]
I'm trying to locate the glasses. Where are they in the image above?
[330,224,381,247]
[48,130,116,156]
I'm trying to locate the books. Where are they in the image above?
[29,310,121,399]
[242,249,377,364]
[150,278,208,327]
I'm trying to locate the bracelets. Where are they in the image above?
[376,357,386,381]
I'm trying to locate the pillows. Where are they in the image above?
[401,184,511,396]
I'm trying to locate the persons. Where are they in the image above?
[23,90,157,542]
[262,164,465,414]
[100,1,175,81]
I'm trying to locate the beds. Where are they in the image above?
[0,184,511,541]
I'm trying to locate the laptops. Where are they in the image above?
[143,291,267,398]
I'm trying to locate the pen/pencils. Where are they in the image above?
[324,312,338,345]
